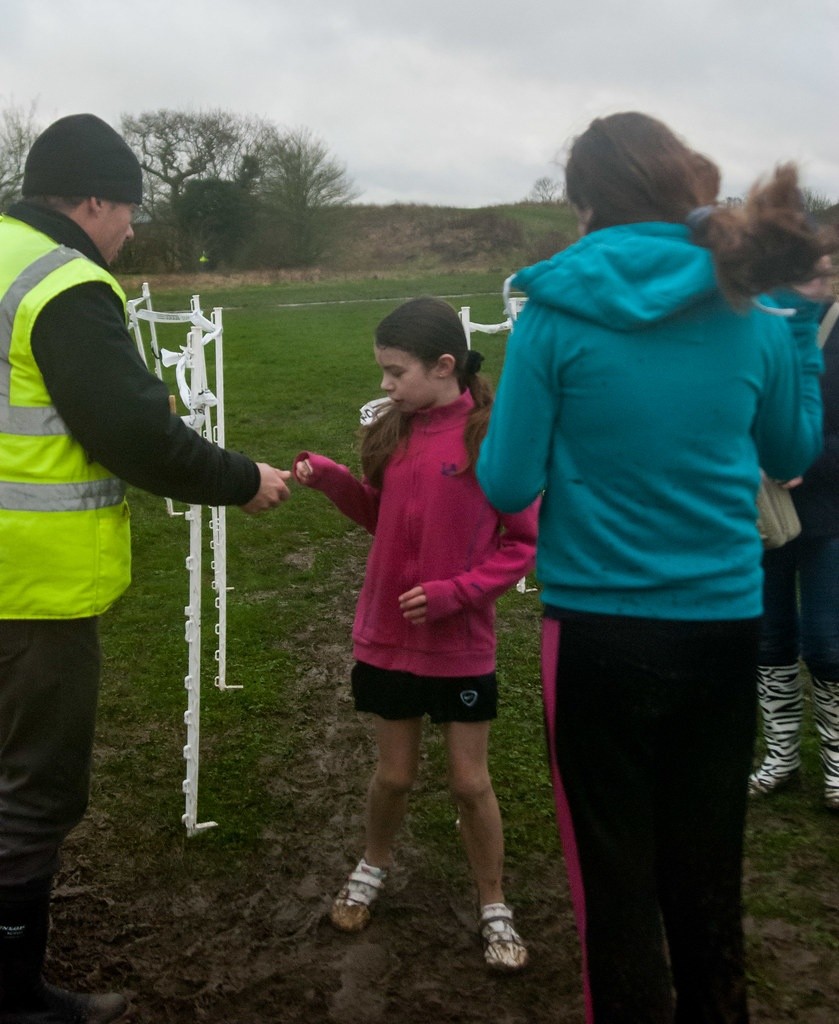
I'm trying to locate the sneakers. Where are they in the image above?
[331,856,389,931]
[481,904,528,973]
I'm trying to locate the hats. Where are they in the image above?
[22,113,143,205]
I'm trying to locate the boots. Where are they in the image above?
[747,661,802,799]
[0,875,130,1024]
[808,673,839,814]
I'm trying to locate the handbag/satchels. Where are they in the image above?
[756,468,802,549]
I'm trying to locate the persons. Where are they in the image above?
[1,108,292,1024]
[294,296,543,977]
[748,201,838,815]
[473,111,822,1024]
